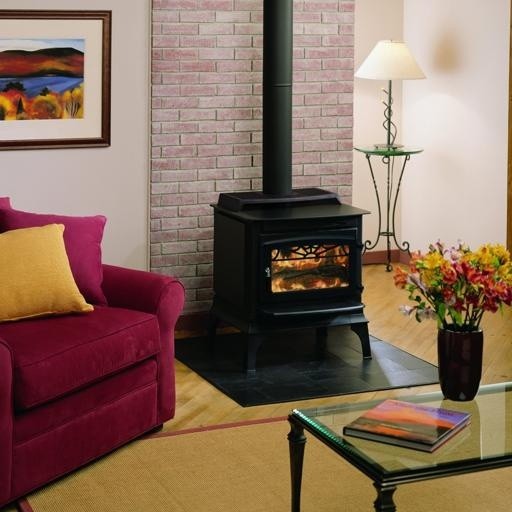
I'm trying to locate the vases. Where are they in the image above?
[438,323,484,401]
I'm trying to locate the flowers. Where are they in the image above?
[393,238,512,330]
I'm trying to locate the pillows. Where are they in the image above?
[0,198,108,305]
[0,223,94,322]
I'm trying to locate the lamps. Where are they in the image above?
[354,41,427,148]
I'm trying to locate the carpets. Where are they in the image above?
[17,416,512,512]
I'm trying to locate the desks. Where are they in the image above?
[353,145,424,272]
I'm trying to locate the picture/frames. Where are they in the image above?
[0,9,112,150]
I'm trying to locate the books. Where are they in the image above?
[342,398,470,454]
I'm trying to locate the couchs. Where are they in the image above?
[0,264,185,508]
